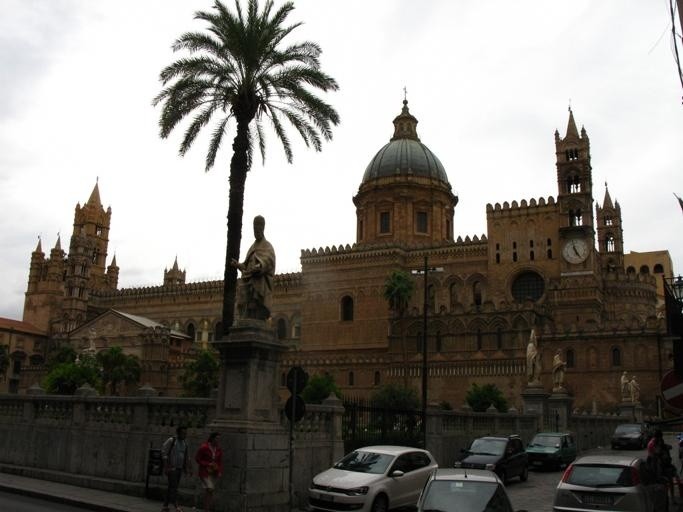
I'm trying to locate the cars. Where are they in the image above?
[552,456,658,512]
[416,469,528,512]
[525,431,576,470]
[611,422,646,449]
[307,444,437,512]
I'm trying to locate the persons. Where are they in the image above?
[230,215,276,320]
[621,371,630,398]
[194,431,225,512]
[630,375,642,403]
[88,327,97,350]
[159,425,193,512]
[525,329,542,384]
[552,349,568,389]
[645,429,665,466]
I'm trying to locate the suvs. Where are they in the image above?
[452,434,529,485]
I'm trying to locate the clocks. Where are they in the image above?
[562,236,590,265]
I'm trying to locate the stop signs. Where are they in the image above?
[660,368,683,412]
